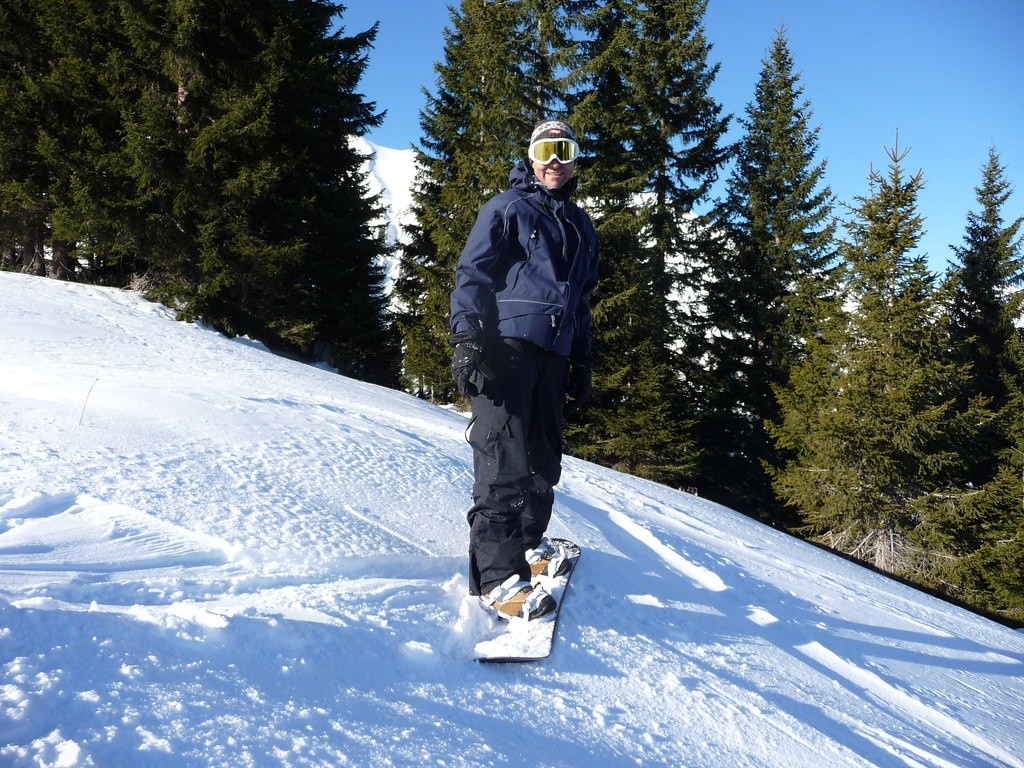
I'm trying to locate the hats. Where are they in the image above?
[532,119,574,142]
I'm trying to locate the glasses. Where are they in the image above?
[528,138,580,165]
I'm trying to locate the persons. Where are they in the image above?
[449,121,599,621]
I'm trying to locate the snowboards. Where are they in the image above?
[471,535,582,664]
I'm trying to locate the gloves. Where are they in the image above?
[451,342,480,398]
[567,366,592,403]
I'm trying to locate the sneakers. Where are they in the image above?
[478,579,557,622]
[529,549,572,577]
